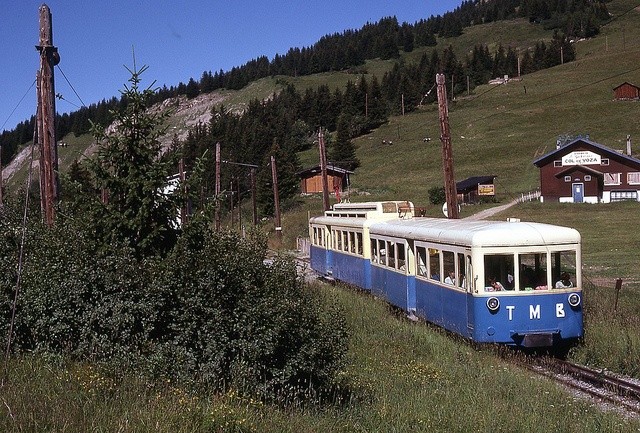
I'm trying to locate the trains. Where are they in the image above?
[328,199,414,221]
[310,219,583,361]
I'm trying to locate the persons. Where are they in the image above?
[554,271,573,288]
[444,266,455,284]
[432,263,440,281]
[486,275,504,291]
